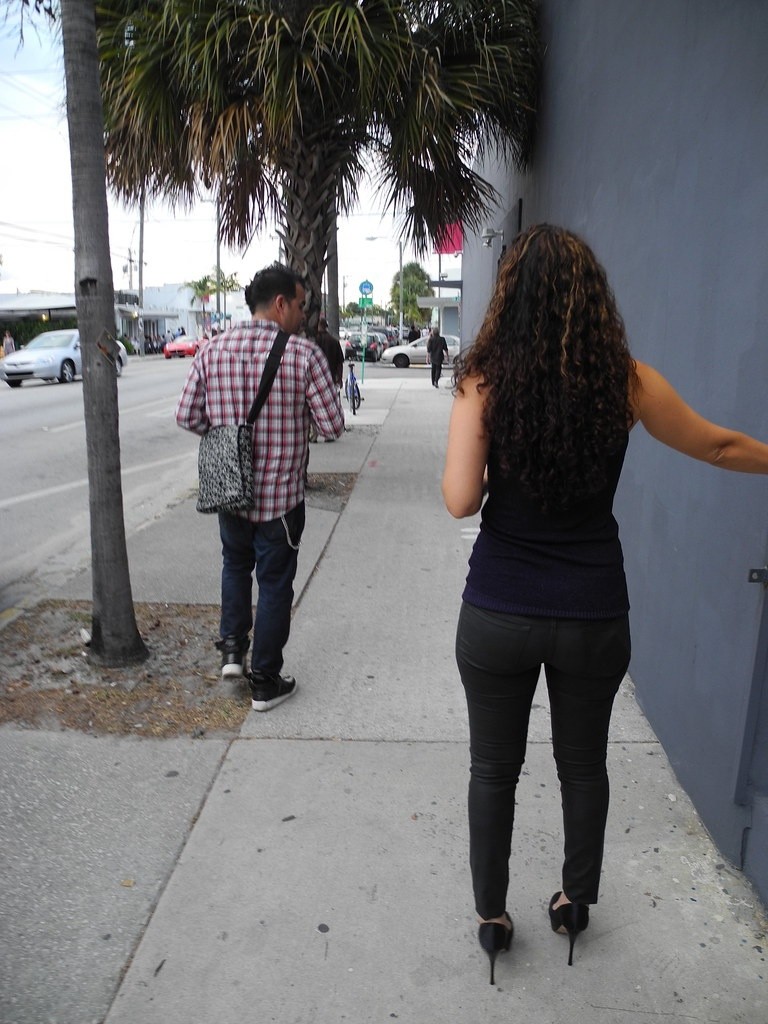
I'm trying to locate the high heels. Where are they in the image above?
[548,891,589,965]
[478,911,514,984]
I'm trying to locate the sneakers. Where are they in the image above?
[249,672,297,711]
[214,636,250,677]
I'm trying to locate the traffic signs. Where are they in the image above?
[359,298,372,308]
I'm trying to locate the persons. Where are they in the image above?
[124,326,185,355]
[426,327,449,388]
[441,226,767,986]
[175,264,344,711]
[407,325,418,343]
[3,330,15,356]
[308,317,344,443]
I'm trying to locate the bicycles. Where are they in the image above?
[342,364,364,415]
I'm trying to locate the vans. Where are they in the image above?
[338,326,352,339]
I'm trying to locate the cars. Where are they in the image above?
[370,327,399,347]
[0,328,127,387]
[344,335,383,361]
[368,333,389,350]
[163,335,206,358]
[380,334,460,369]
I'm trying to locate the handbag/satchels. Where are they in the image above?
[196,424,255,514]
